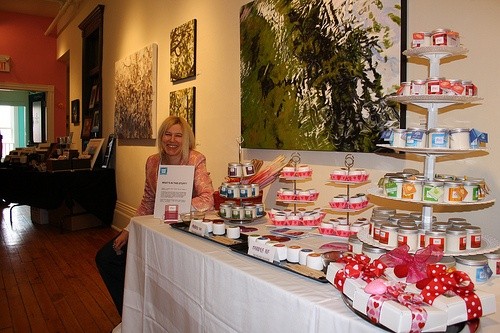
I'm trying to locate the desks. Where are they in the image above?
[112,213,394,333]
[0,165,115,226]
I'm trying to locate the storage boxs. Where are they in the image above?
[327,262,497,332]
[31,199,103,231]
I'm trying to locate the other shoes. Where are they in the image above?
[112,322,122,333]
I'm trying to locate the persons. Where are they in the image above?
[94,115,216,333]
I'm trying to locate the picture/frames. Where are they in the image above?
[102,134,116,168]
[84,138,105,169]
[71,99,80,123]
[80,85,101,139]
